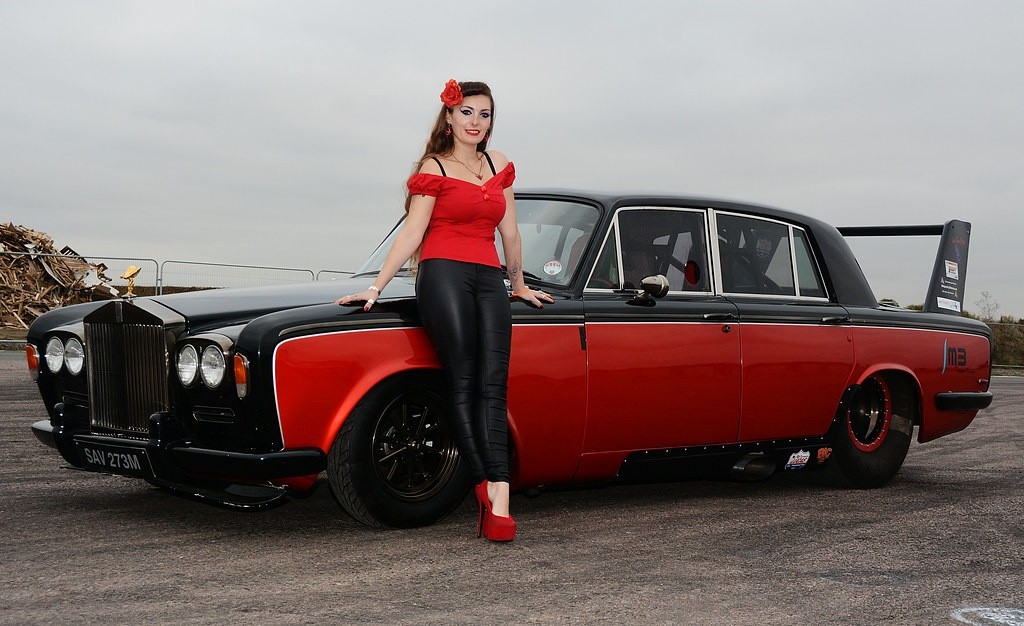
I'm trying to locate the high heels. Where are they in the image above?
[475,479,516,541]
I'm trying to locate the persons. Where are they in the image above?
[121,265,141,295]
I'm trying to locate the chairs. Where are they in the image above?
[564,235,609,289]
[682,239,731,292]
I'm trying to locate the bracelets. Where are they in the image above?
[367,285,381,296]
[512,284,529,296]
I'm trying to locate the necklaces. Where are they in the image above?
[447,142,485,181]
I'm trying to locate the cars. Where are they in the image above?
[24,184,995,534]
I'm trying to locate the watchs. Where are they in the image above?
[334,79,556,543]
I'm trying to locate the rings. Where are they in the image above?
[538,290,548,300]
[367,298,375,305]
[346,293,358,298]
